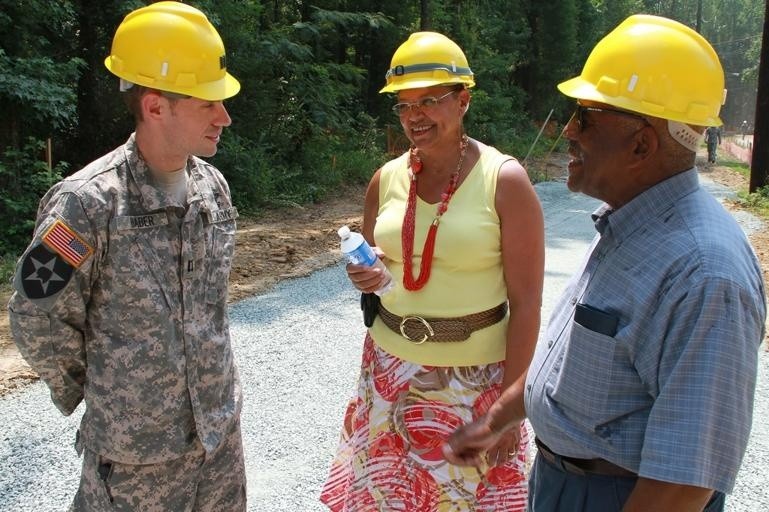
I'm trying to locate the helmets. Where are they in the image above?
[379,32,475,94]
[556,15,724,127]
[104,2,241,102]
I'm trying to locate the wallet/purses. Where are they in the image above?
[573,303,620,337]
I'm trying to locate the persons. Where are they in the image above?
[5,0,252,512]
[322,29,546,511]
[740,120,749,140]
[703,125,721,163]
[437,14,766,512]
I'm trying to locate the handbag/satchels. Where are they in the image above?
[361,291,379,327]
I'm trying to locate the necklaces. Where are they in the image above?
[393,134,472,289]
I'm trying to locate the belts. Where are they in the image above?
[535,435,635,476]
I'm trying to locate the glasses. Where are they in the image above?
[392,91,456,117]
[576,102,650,131]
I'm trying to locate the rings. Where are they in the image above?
[445,444,455,453]
[508,452,516,457]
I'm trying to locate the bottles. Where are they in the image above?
[338,225,397,298]
